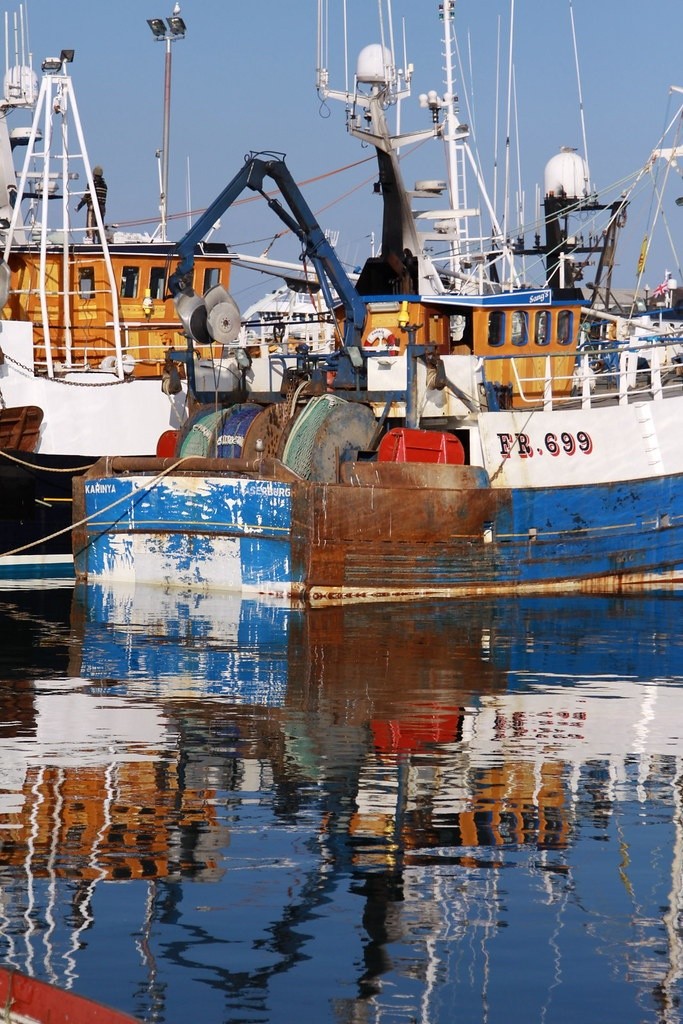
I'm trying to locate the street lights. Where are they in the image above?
[144,17,185,241]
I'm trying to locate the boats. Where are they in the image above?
[0,0,683,609]
